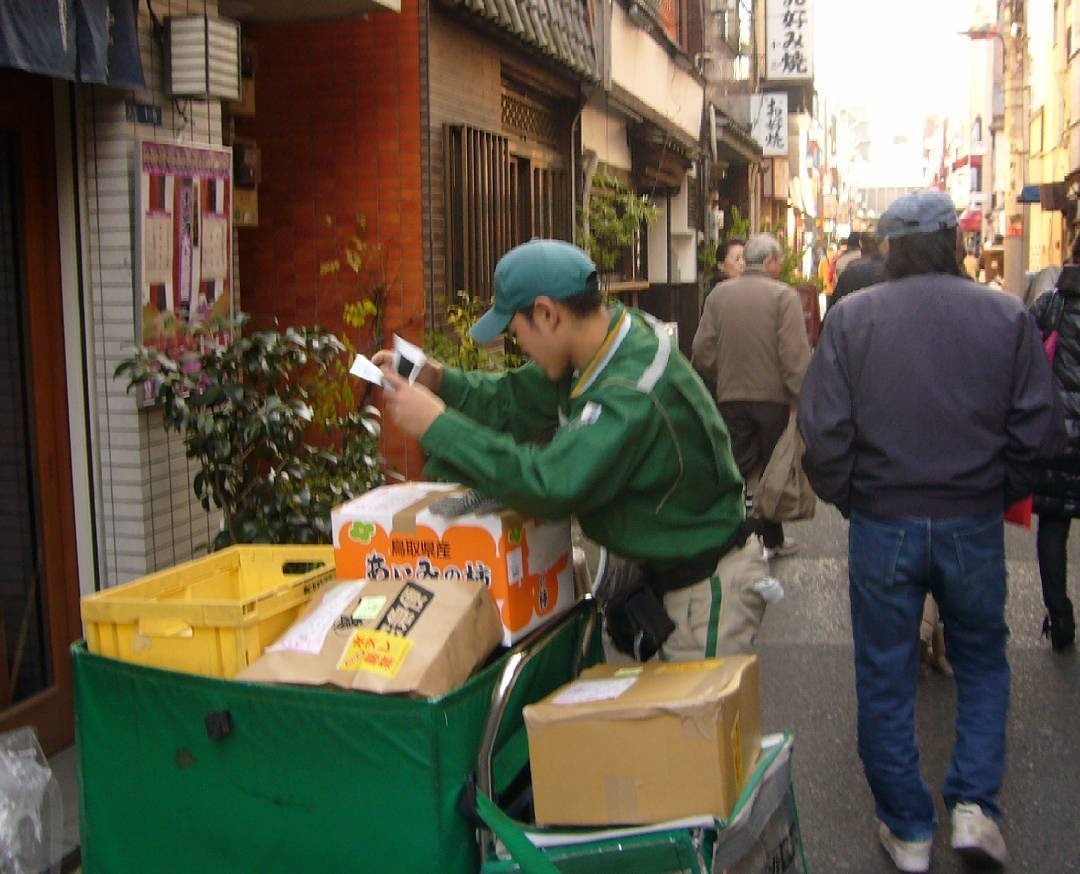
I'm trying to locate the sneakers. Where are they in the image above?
[951,803,1007,871]
[880,823,932,871]
[764,537,797,560]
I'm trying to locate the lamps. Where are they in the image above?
[161,14,243,103]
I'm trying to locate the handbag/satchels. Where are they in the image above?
[602,522,756,662]
[1040,290,1066,365]
[752,399,816,520]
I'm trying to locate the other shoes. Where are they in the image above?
[1044,596,1076,645]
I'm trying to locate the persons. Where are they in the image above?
[691,189,1080,874]
[364,238,767,657]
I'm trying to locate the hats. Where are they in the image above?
[886,192,956,239]
[468,239,598,342]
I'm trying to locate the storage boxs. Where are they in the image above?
[70,481,809,874]
